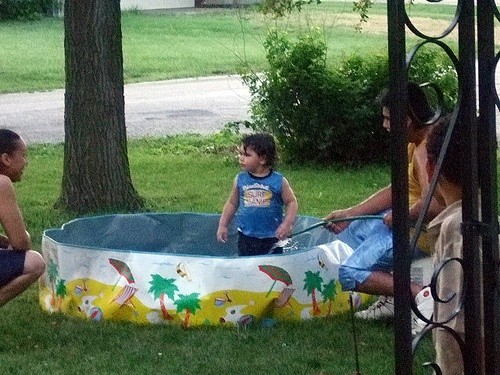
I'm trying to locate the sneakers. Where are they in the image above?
[411,287,434,336]
[354,295,394,320]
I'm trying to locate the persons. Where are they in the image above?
[426,111,498,375]
[0,128,46,308]
[216,133,299,255]
[321,80,446,338]
